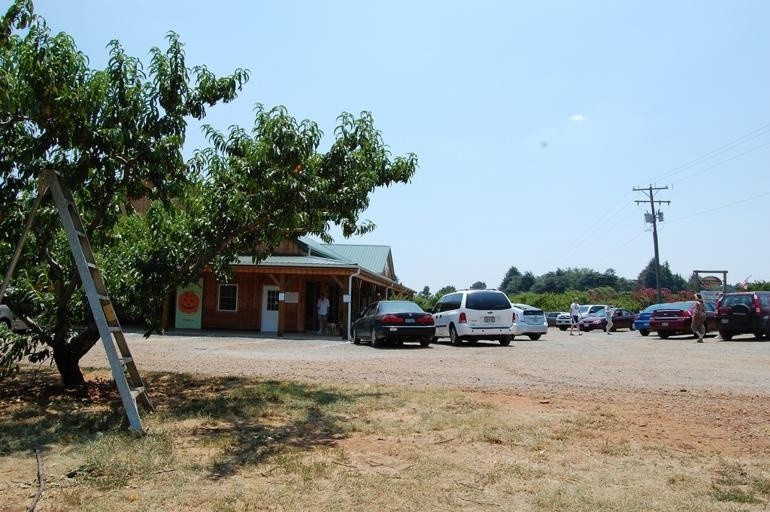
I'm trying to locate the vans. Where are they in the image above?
[431,287,520,347]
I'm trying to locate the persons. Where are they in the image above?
[314,291,330,335]
[691,292,707,342]
[605,301,614,334]
[568,296,584,336]
[607,301,615,318]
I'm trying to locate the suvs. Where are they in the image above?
[716,289,770,342]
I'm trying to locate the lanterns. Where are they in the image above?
[178,288,200,314]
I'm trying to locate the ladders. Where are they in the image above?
[0,170,154,440]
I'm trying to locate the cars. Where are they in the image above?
[350,299,437,349]
[511,301,550,343]
[545,299,718,340]
